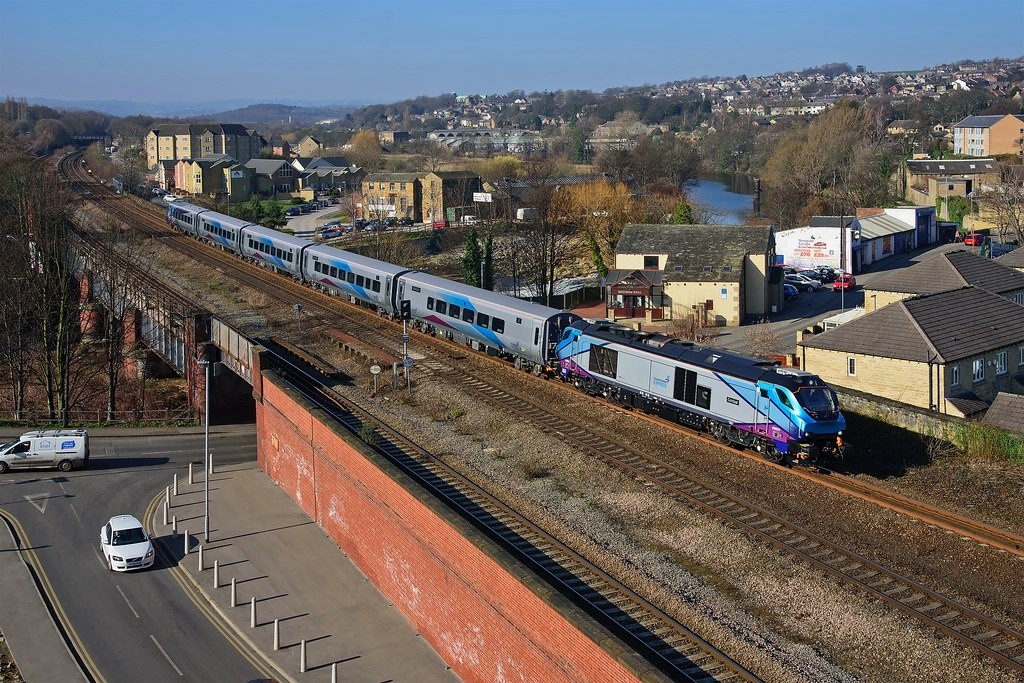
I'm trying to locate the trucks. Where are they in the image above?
[0,427,90,472]
[516,208,544,222]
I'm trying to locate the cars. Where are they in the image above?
[593,209,610,216]
[833,274,856,292]
[775,265,845,301]
[965,234,991,246]
[99,514,156,572]
[286,198,338,215]
[321,216,415,240]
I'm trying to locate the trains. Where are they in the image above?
[165,200,854,464]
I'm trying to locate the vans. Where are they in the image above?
[461,215,481,225]
